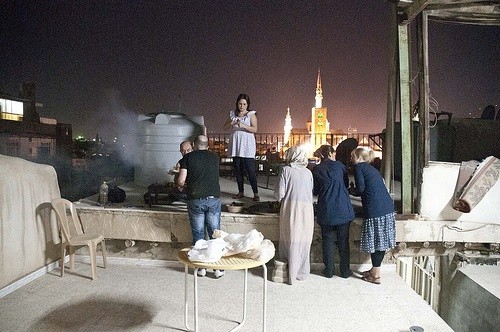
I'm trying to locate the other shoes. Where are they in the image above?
[254,195,259,200]
[343,271,353,278]
[234,194,244,199]
[197,268,206,277]
[214,269,225,278]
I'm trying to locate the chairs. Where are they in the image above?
[50,197,109,280]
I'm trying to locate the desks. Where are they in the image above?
[177,246,268,332]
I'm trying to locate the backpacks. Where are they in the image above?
[143,183,174,205]
[98,185,126,202]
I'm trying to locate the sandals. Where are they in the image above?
[362,275,381,284]
[360,271,372,277]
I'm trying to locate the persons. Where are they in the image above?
[312,145,353,278]
[275,147,314,284]
[349,148,394,283]
[168,141,194,202]
[176,135,225,278]
[223,94,260,201]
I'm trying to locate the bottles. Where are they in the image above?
[99,181,108,206]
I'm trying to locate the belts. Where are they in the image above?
[195,196,219,199]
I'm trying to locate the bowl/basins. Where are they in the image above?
[226,204,243,213]
